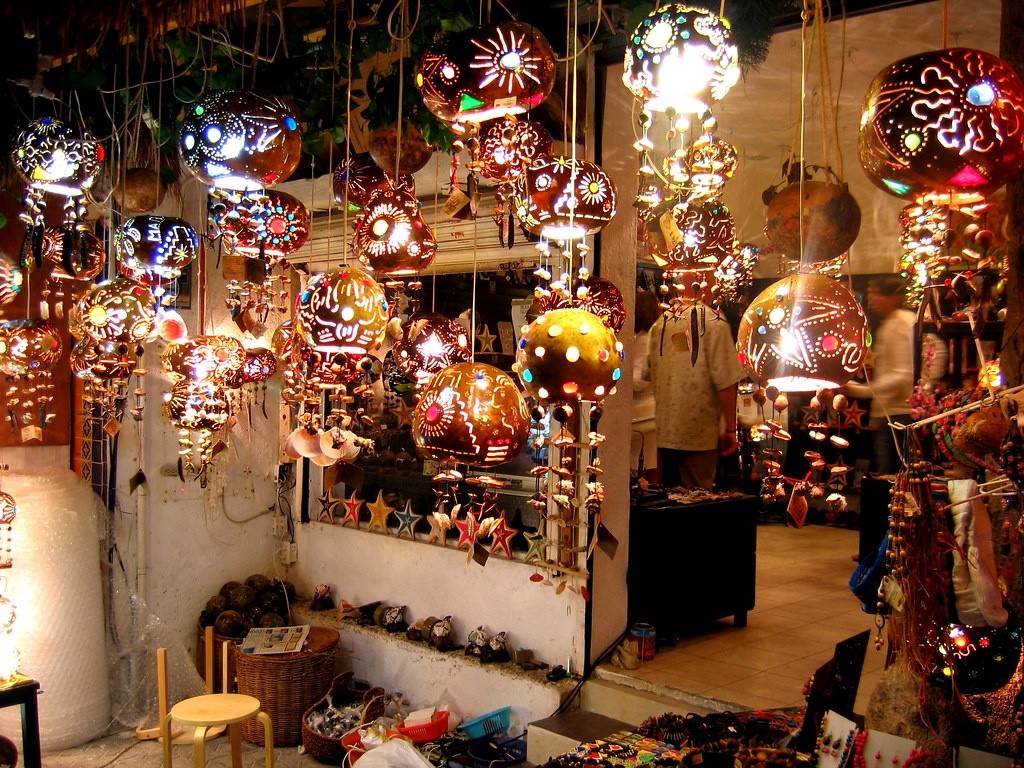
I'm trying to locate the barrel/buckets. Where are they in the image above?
[342,729,411,768]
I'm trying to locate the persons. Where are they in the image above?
[837,275,928,561]
[633,291,662,402]
[641,283,747,492]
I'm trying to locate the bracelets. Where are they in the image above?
[724,428,738,433]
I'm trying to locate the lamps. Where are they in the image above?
[0,491,21,685]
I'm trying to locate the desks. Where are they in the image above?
[633,494,763,644]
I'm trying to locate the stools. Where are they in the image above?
[0,671,41,768]
[162,692,275,768]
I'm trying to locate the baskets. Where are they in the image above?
[397,710,450,743]
[197,619,245,693]
[457,707,511,739]
[302,669,385,766]
[235,648,337,747]
[342,730,401,768]
